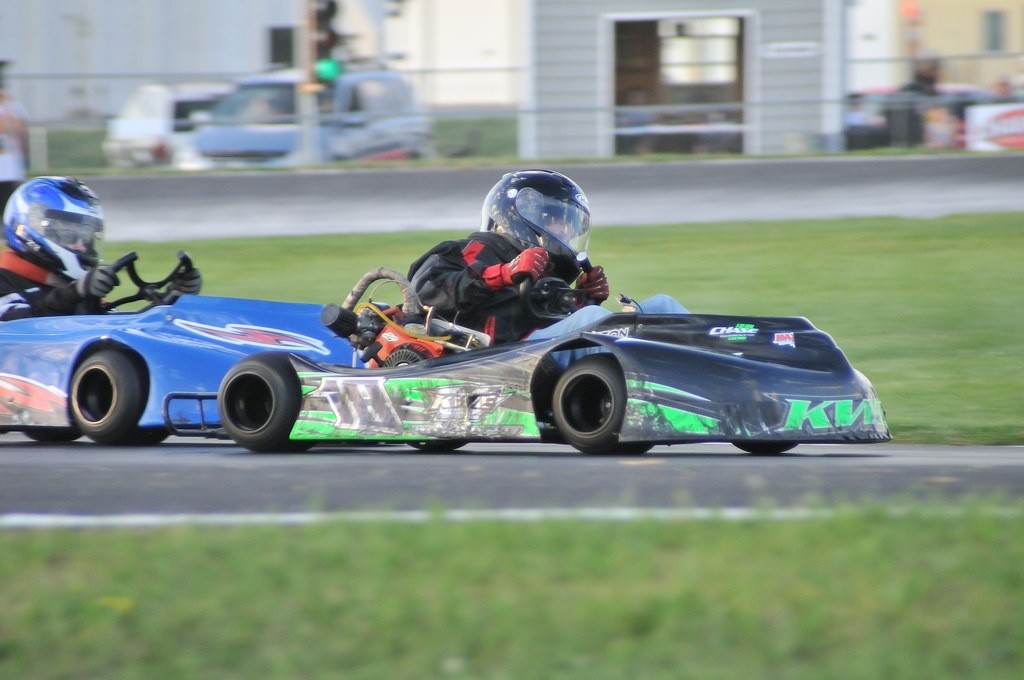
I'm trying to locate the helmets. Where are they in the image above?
[2,175,106,281]
[481,168,591,267]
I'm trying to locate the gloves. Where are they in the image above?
[169,267,203,293]
[575,265,610,308]
[482,246,549,293]
[75,264,120,301]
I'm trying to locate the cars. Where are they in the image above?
[102,83,234,168]
[842,85,982,118]
[194,69,434,170]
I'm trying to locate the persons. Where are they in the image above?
[410,169,609,347]
[843,48,1013,147]
[0,176,202,321]
[0,59,32,244]
